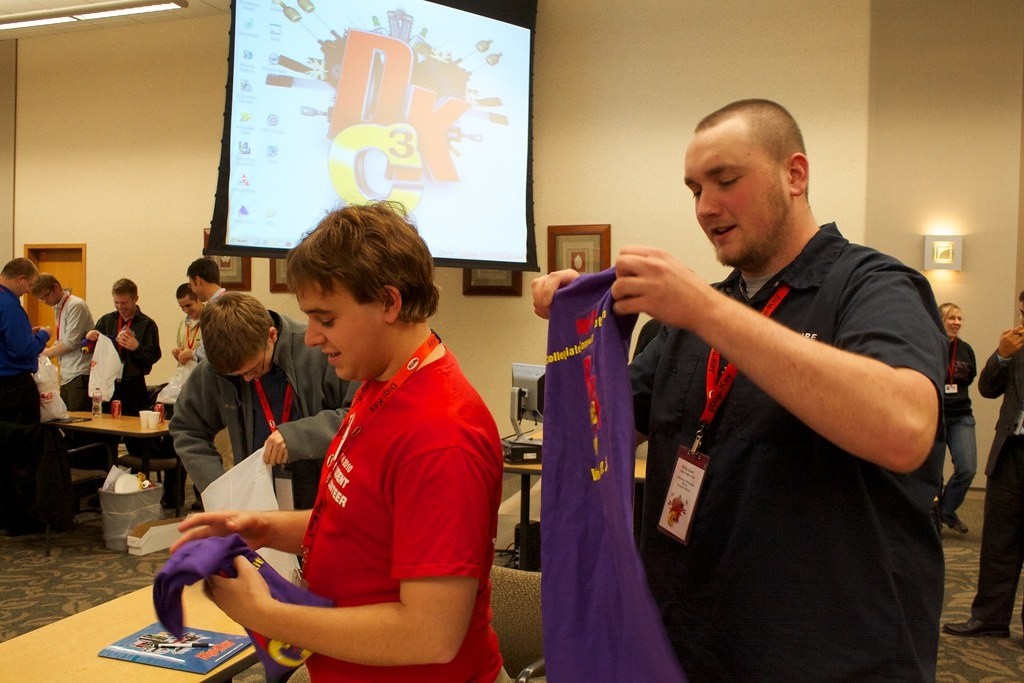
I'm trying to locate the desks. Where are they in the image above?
[498,430,648,568]
[39,410,171,480]
[0,577,260,683]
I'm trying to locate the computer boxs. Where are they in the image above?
[500,440,546,464]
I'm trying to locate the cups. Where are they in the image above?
[148,412,160,428]
[140,410,153,428]
[115,325,135,345]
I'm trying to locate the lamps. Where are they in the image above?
[0,0,190,31]
[924,235,963,270]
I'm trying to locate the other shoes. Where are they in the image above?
[192,497,204,509]
[160,496,185,512]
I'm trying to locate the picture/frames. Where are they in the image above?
[269,258,293,292]
[204,227,252,290]
[463,267,523,297]
[547,225,612,278]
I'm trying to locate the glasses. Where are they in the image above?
[36,284,55,302]
[227,334,270,377]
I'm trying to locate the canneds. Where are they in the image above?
[141,480,156,488]
[111,400,121,419]
[154,404,164,423]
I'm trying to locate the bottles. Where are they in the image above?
[92,388,102,417]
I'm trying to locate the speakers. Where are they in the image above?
[513,520,541,571]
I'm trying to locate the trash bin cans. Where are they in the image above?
[97,483,162,551]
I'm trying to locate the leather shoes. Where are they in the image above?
[943,617,1009,638]
[942,511,970,532]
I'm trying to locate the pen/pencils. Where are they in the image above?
[153,643,215,648]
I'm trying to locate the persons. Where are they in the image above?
[0,257,234,537]
[168,202,511,683]
[935,302,977,536]
[169,292,363,533]
[943,291,1024,635]
[531,98,948,683]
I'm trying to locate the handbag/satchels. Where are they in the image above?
[200,449,303,586]
[88,334,122,401]
[156,357,202,405]
[31,353,67,422]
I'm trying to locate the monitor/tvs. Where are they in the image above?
[507,362,547,445]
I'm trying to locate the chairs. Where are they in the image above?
[33,381,181,557]
[488,566,547,683]
